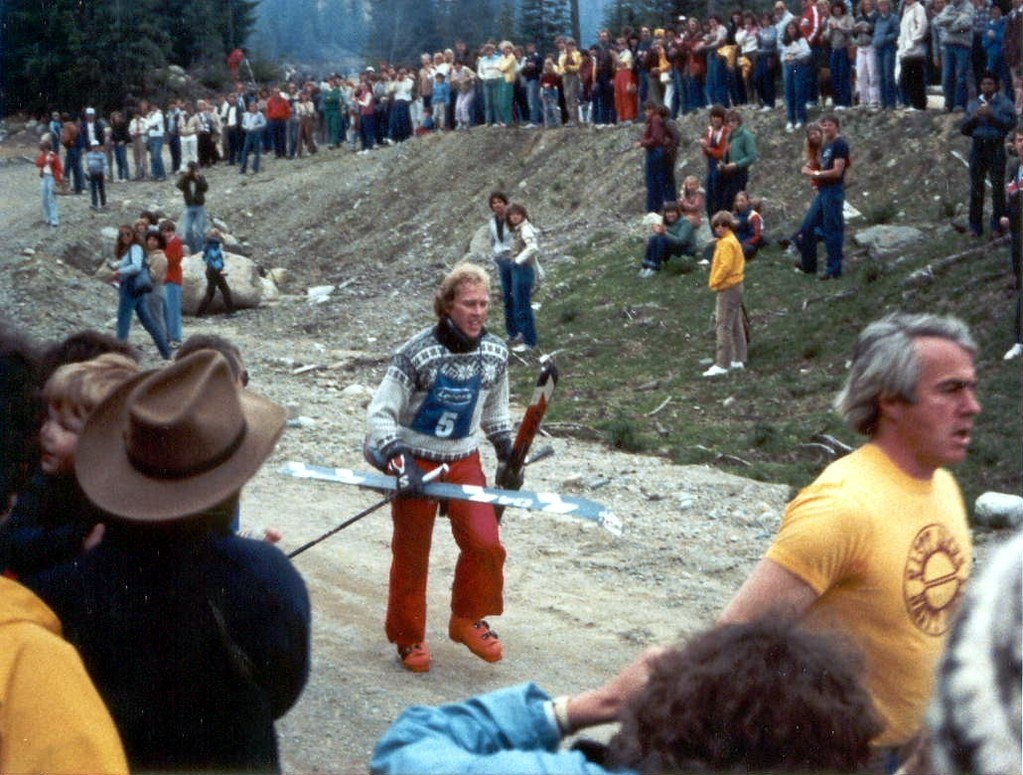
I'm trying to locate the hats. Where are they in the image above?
[91,140,101,147]
[86,107,95,115]
[72,349,288,524]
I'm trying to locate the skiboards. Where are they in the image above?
[276,367,629,539]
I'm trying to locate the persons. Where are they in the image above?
[103,210,186,362]
[37,141,63,227]
[82,138,109,213]
[713,312,981,775]
[35,0,1023,197]
[960,73,1023,240]
[0,324,312,775]
[364,265,527,673]
[789,113,850,282]
[195,226,242,321]
[696,104,758,223]
[633,99,682,212]
[700,211,750,378]
[1001,126,1023,362]
[370,599,882,775]
[634,174,764,281]
[486,192,544,351]
[174,161,209,254]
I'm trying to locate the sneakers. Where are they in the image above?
[449,614,504,662]
[395,643,431,673]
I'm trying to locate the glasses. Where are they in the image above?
[713,221,731,227]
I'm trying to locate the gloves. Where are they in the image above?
[494,439,525,492]
[385,451,426,499]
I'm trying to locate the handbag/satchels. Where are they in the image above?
[128,244,154,296]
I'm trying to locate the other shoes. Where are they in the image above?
[224,313,239,318]
[504,333,538,352]
[638,266,659,278]
[355,136,397,156]
[1002,342,1023,361]
[427,116,642,132]
[728,361,745,368]
[963,230,978,237]
[702,365,727,377]
[991,230,1004,239]
[794,262,804,271]
[695,259,710,265]
